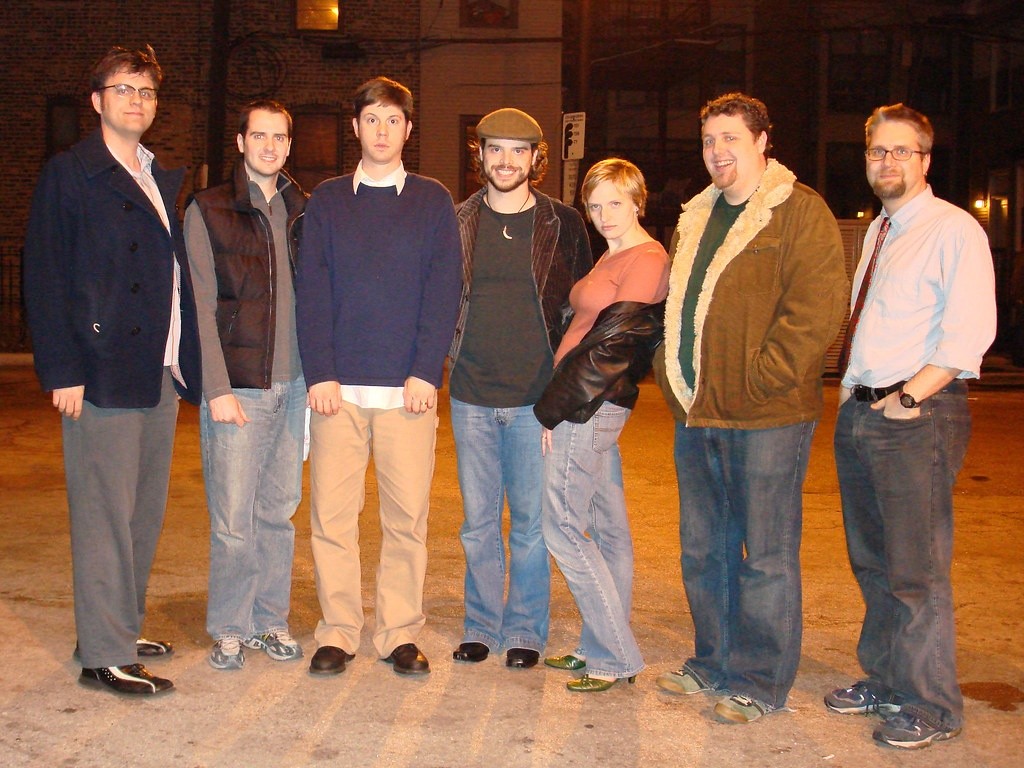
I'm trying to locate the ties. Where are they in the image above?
[837,217,891,379]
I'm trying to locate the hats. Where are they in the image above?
[475,108,543,143]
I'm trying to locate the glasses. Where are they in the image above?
[864,147,926,160]
[98,84,158,100]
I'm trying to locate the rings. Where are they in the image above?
[542,436,547,439]
[421,402,427,405]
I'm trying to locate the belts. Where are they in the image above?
[855,380,906,403]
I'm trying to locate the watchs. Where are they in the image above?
[899,383,923,408]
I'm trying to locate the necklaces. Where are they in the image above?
[487,187,530,212]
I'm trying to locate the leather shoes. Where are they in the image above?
[309,646,355,675]
[507,648,540,667]
[453,642,489,664]
[384,644,431,674]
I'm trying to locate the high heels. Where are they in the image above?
[545,655,586,670]
[567,674,635,692]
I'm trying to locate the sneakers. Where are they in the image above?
[74,639,175,661]
[871,711,960,749]
[714,694,773,723]
[243,630,304,660]
[79,663,175,697]
[824,681,903,717]
[656,668,711,695]
[209,637,244,669]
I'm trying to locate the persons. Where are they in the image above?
[830,104,999,748]
[19,45,202,696]
[532,159,672,693]
[445,108,595,668]
[651,97,847,725]
[293,77,465,676]
[182,103,317,668]
